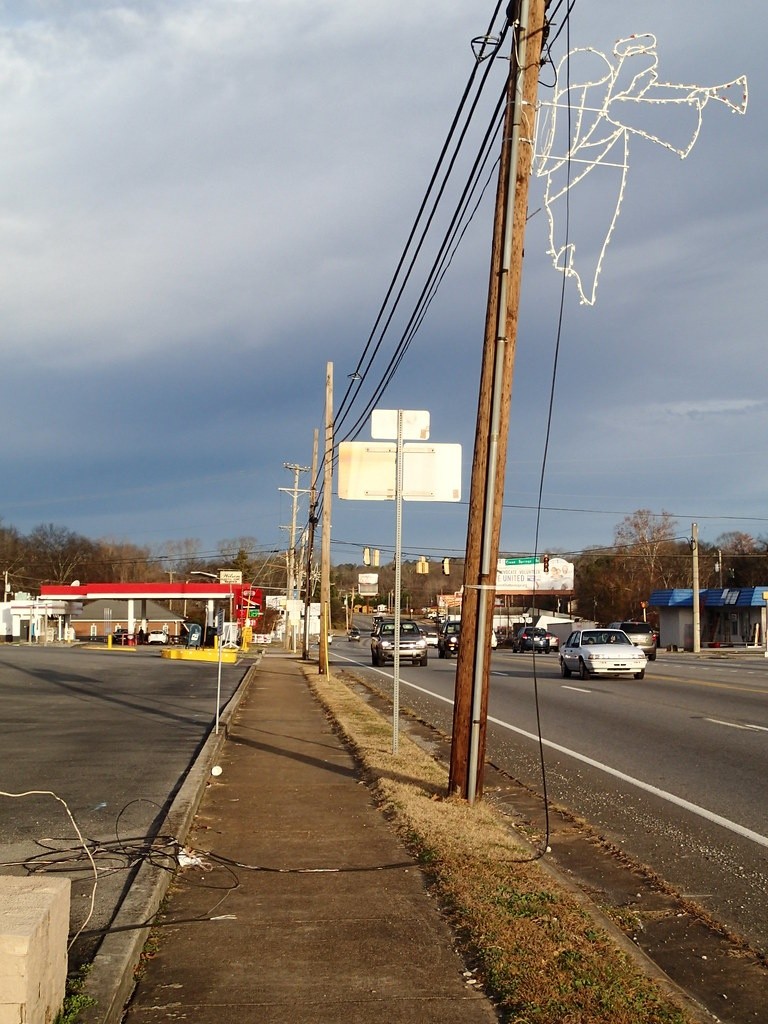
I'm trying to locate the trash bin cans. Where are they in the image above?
[186,624,201,650]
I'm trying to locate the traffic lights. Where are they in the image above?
[543,557,549,573]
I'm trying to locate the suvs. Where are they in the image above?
[606,622,657,660]
[371,620,428,668]
[513,627,550,655]
[437,620,462,659]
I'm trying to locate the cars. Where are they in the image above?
[425,632,440,648]
[113,628,128,643]
[348,631,361,642]
[558,629,647,680]
[545,631,560,652]
[317,632,333,646]
[147,629,169,645]
[490,629,498,651]
